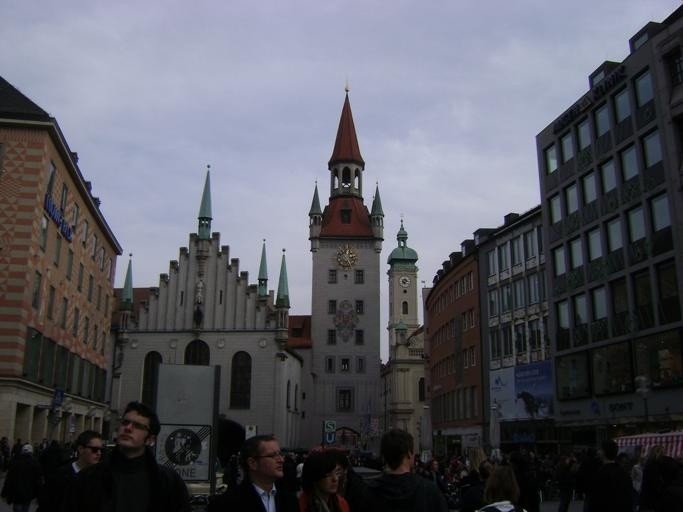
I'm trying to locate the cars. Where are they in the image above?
[352,465,380,487]
[104,443,116,452]
[185,469,226,504]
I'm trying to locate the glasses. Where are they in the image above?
[318,469,344,481]
[82,442,107,456]
[119,417,152,436]
[253,452,287,459]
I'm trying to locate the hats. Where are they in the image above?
[20,443,34,457]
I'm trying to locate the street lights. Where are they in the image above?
[635,374,652,432]
